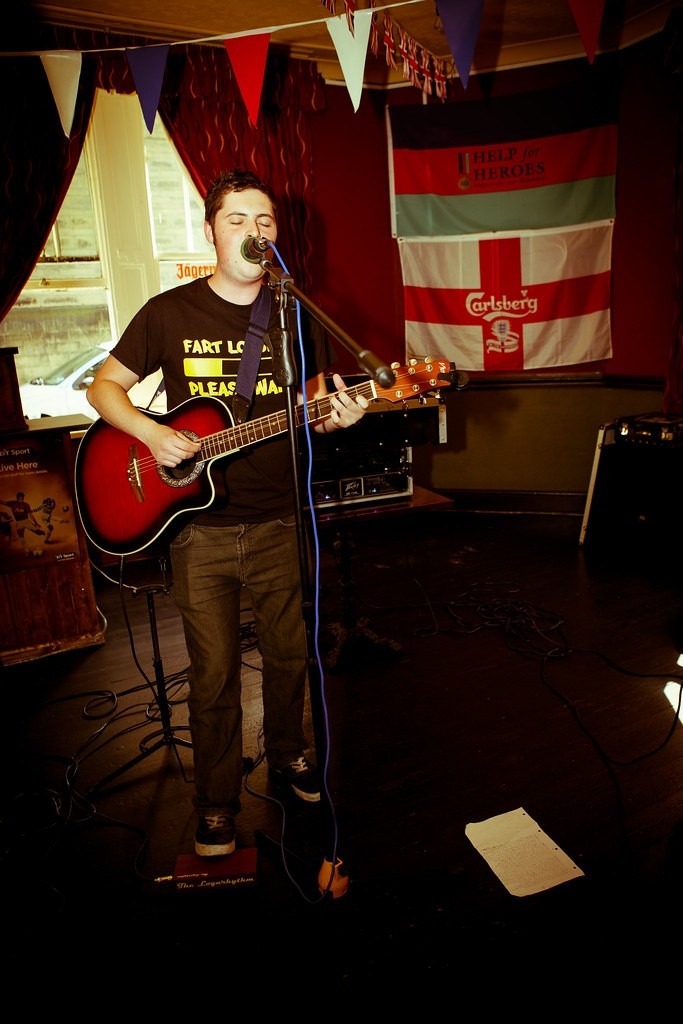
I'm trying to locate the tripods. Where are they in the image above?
[86,590,253,798]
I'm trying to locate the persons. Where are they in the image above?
[88,176,370,858]
[0,492,56,546]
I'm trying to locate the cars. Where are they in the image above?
[19,339,168,421]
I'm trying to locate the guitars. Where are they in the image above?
[72,355,471,559]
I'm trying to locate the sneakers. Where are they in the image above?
[268,754,321,802]
[193,813,238,858]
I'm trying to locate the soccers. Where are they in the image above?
[63,505,70,512]
[33,546,44,557]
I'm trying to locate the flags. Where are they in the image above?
[391,121,617,374]
[382,12,398,72]
[344,0,356,38]
[368,0,379,59]
[321,0,337,15]
[400,31,449,101]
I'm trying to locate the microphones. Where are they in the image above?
[241,237,270,263]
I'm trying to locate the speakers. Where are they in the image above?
[595,441,683,601]
